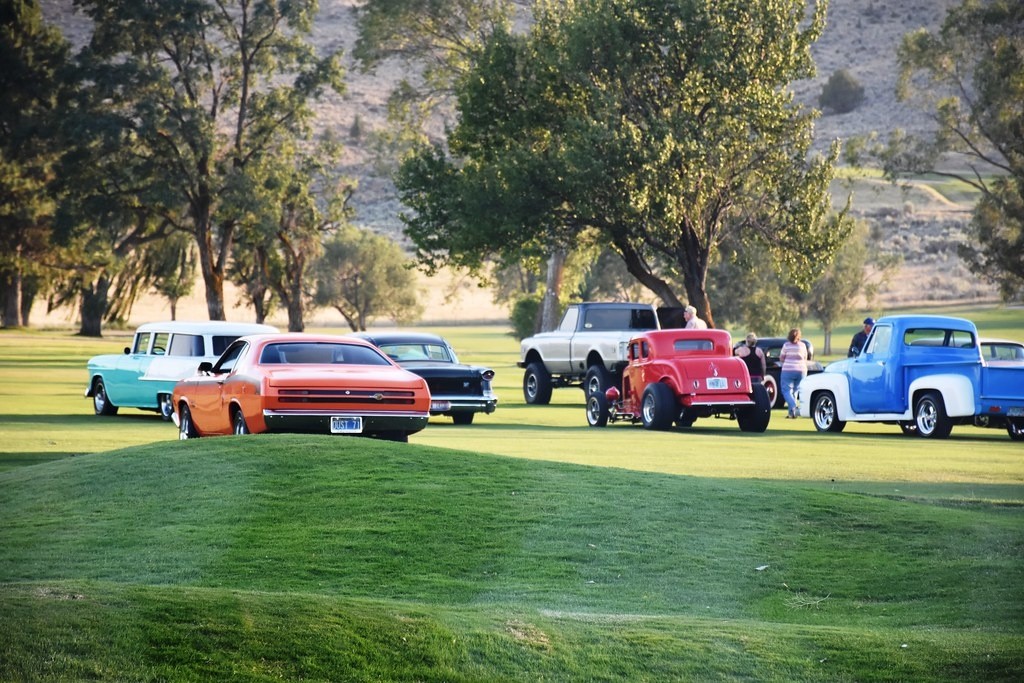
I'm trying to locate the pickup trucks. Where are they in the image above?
[797,312,1024,440]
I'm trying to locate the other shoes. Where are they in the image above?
[792,407,798,418]
[785,416,794,419]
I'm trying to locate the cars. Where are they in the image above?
[171,332,432,443]
[84,321,290,421]
[585,327,771,434]
[910,337,1024,365]
[731,337,826,409]
[331,330,498,426]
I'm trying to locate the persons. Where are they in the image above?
[685,307,707,331]
[730,333,766,419]
[780,329,808,419]
[848,317,874,358]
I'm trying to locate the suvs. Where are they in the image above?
[516,301,690,407]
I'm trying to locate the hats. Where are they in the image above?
[864,317,875,326]
